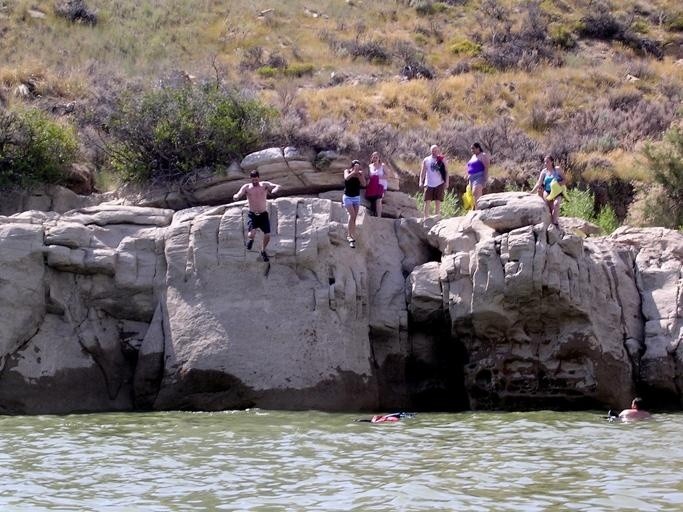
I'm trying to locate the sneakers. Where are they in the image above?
[246,239,254,250]
[347,235,356,248]
[261,250,269,262]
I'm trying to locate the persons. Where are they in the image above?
[367,151,388,218]
[418,145,450,219]
[523,155,568,228]
[341,159,367,248]
[467,142,490,210]
[233,170,282,262]
[537,183,545,198]
[619,396,651,420]
